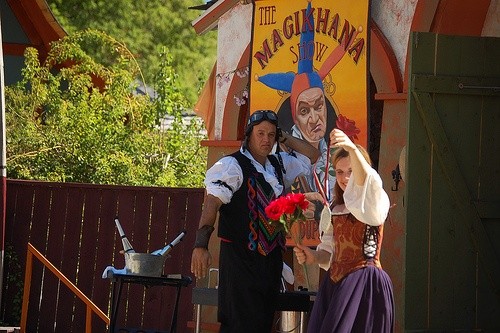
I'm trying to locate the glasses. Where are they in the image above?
[246,111,277,126]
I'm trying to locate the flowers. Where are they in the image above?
[266,193,313,293]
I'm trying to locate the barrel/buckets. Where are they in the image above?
[120,250,168,277]
[274,310,312,333]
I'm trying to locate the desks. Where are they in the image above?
[108,270,193,333]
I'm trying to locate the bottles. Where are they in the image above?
[298,286,310,302]
[150,231,187,256]
[115,218,134,253]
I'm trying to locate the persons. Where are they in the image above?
[191,109,286,333]
[294,128,394,333]
